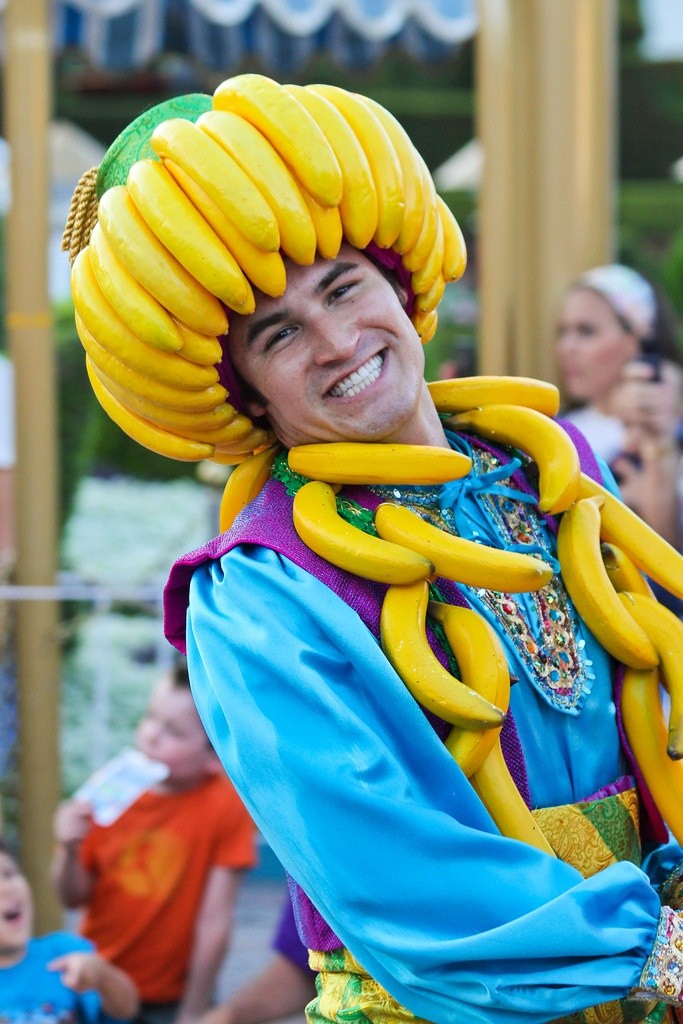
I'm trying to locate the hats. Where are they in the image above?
[65,72,469,464]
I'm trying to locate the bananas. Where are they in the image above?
[213,374,682,865]
[70,74,469,466]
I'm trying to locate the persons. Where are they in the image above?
[199,895,318,1023]
[52,661,257,1024]
[556,260,683,621]
[0,841,139,1024]
[61,73,683,1024]
